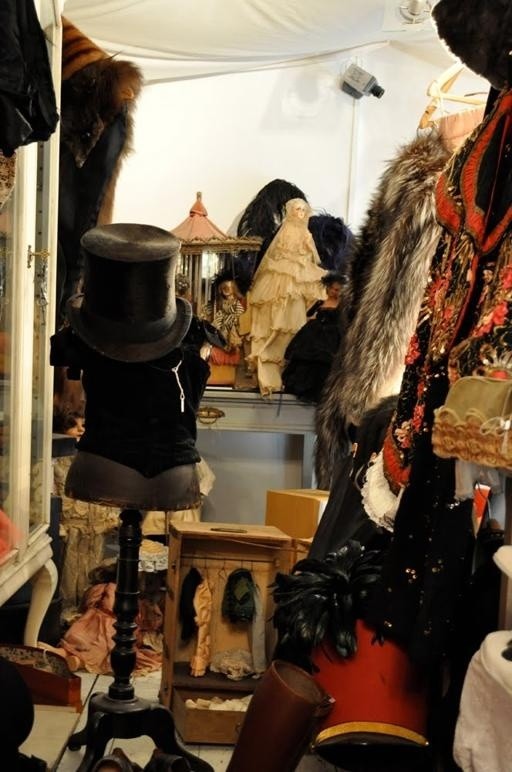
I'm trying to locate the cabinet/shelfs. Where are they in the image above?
[158,519,298,746]
[0,0,80,672]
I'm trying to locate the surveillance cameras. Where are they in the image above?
[339,63,385,100]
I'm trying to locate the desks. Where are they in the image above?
[197,387,329,489]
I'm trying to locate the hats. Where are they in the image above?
[67,224,192,364]
[299,618,435,771]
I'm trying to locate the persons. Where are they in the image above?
[51,398,123,629]
[281,270,349,409]
[242,196,330,402]
[51,221,212,510]
